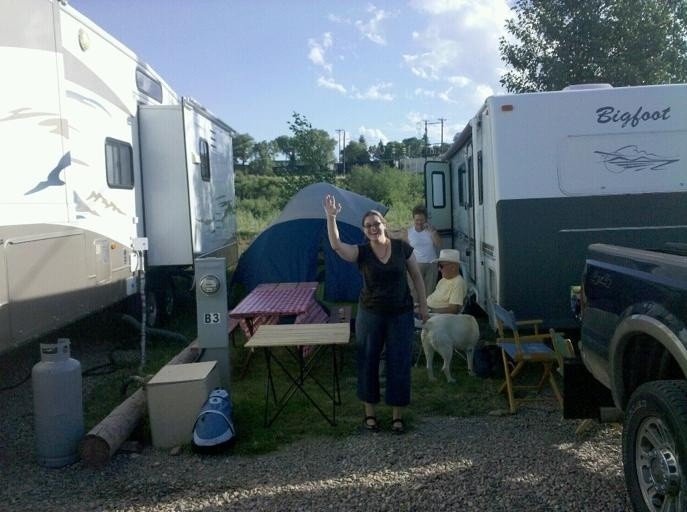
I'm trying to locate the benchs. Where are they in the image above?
[192,309,239,363]
[329,306,351,324]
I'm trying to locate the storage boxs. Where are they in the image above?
[145,361,221,449]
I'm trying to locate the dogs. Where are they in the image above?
[414,312,481,384]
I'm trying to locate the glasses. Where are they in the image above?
[439,264,451,269]
[363,223,381,228]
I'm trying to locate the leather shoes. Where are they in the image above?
[390,419,403,433]
[364,416,379,432]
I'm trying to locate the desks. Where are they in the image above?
[244,323,351,428]
[229,282,319,380]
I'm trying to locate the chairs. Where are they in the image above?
[414,286,475,367]
[492,299,565,414]
[549,328,576,372]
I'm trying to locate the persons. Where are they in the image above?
[413,249,467,320]
[322,194,429,434]
[400,204,443,303]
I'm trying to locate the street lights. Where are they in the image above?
[342,128,346,174]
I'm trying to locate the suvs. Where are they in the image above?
[576,237,687,512]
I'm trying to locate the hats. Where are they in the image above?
[430,249,465,264]
